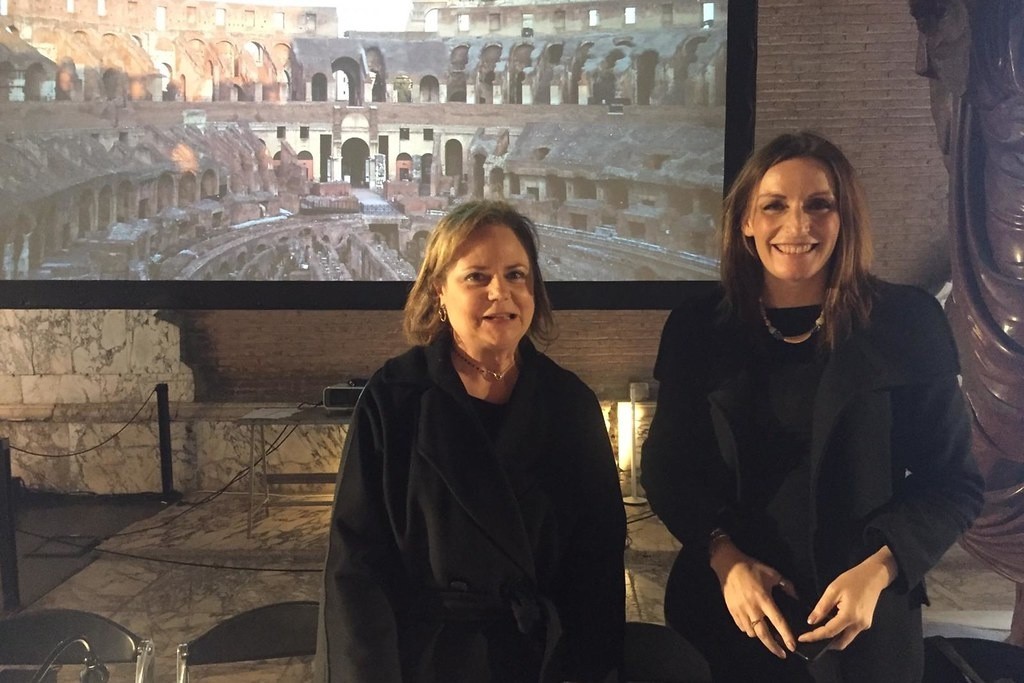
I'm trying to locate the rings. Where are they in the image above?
[751,619,760,626]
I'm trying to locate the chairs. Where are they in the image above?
[173,598,326,683]
[1,606,157,683]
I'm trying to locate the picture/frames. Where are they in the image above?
[0,1,768,319]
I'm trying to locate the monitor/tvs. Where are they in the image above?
[0,0,759,308]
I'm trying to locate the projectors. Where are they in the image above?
[323,383,365,409]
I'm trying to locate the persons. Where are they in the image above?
[640,130,985,681]
[329,198,629,683]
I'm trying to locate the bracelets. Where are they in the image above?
[703,528,727,543]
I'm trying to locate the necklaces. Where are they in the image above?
[757,279,832,343]
[450,344,519,380]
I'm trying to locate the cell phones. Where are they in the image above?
[762,587,842,661]
